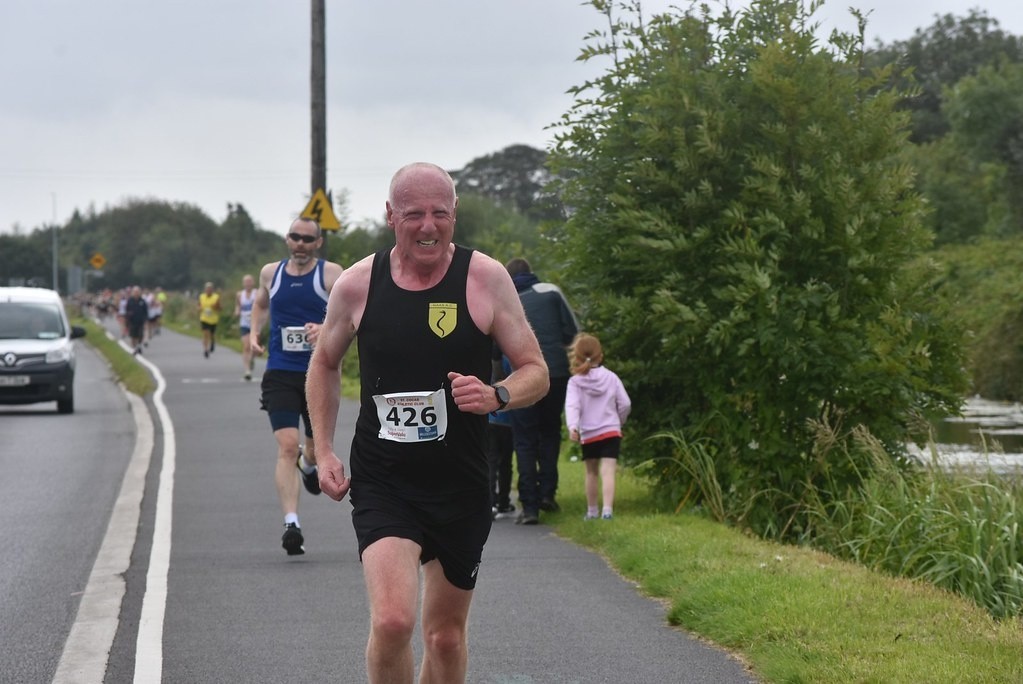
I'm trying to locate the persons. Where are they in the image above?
[198,281,222,357]
[565,334,631,521]
[250,218,343,555]
[303,162,549,684]
[488,356,516,517]
[234,275,258,382]
[84,285,164,348]
[491,257,580,525]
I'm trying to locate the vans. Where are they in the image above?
[0,286,86,413]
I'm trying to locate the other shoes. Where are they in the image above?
[513,511,538,525]
[245,372,251,379]
[540,497,559,512]
[584,515,600,520]
[603,513,612,519]
[492,506,519,519]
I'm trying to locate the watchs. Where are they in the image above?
[490,385,510,417]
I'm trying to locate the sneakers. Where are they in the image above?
[295,444,321,495]
[283,521,305,555]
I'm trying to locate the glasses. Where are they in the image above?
[288,233,317,243]
